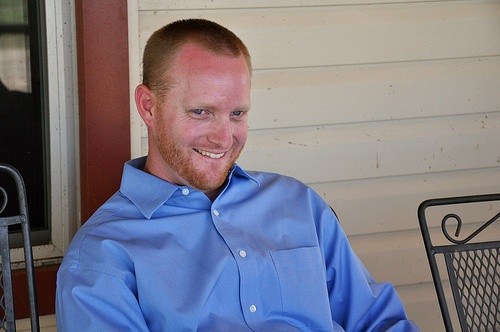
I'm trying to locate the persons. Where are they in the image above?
[54,19,415,331]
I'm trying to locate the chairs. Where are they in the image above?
[0,162,42,332]
[417,191,500,332]
